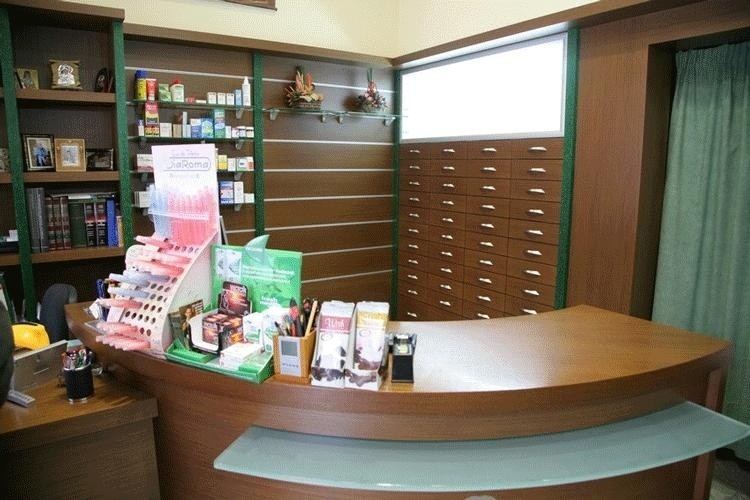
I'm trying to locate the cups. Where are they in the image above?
[61,361,97,403]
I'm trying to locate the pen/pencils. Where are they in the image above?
[62,348,95,370]
[274,296,322,337]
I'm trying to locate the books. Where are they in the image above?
[314,299,390,371]
[26,186,124,256]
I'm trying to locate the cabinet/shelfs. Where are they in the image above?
[2,371,163,500]
[396,135,564,323]
[2,1,259,346]
[263,105,398,127]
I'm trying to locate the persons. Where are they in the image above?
[182,306,196,337]
